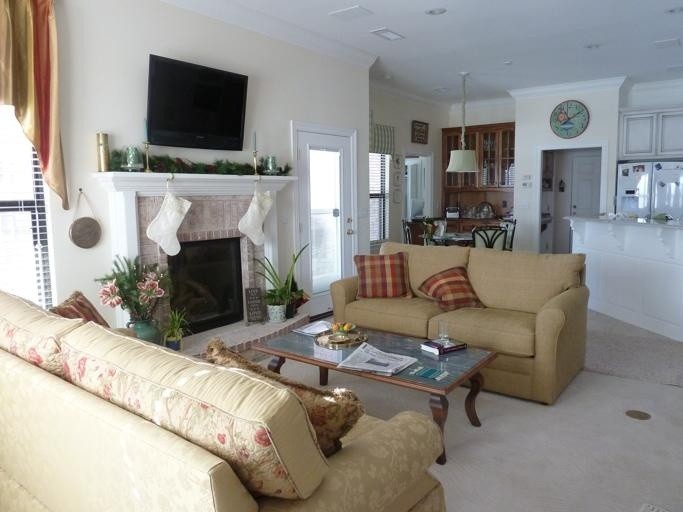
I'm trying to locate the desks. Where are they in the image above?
[418,232,472,248]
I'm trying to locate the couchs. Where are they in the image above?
[0,328,443,512]
[330,242,590,405]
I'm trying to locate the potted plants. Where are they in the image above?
[263,287,289,323]
[160,307,199,353]
[254,243,314,318]
[285,273,310,314]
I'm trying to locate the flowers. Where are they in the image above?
[93,254,187,322]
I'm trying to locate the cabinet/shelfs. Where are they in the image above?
[562,216,683,342]
[91,172,298,327]
[619,105,683,160]
[412,122,515,246]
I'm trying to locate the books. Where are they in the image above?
[419,337,468,355]
[292,320,333,338]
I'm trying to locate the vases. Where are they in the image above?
[126,320,159,345]
[424,238,436,246]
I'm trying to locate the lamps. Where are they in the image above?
[444,71,481,172]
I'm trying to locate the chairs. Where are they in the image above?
[499,219,517,249]
[402,218,411,244]
[472,226,508,251]
[423,217,448,244]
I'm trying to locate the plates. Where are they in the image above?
[327,333,350,342]
[314,329,368,348]
[267,317,286,324]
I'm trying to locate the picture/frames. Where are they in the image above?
[411,120,429,144]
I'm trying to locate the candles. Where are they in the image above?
[142,116,148,140]
[251,130,256,150]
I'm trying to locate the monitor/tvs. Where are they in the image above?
[145,53,249,152]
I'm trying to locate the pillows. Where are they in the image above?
[354,252,413,301]
[0,291,85,381]
[208,336,365,457]
[52,290,108,329]
[417,267,487,311]
[61,324,330,498]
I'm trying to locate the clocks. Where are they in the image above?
[550,100,589,139]
